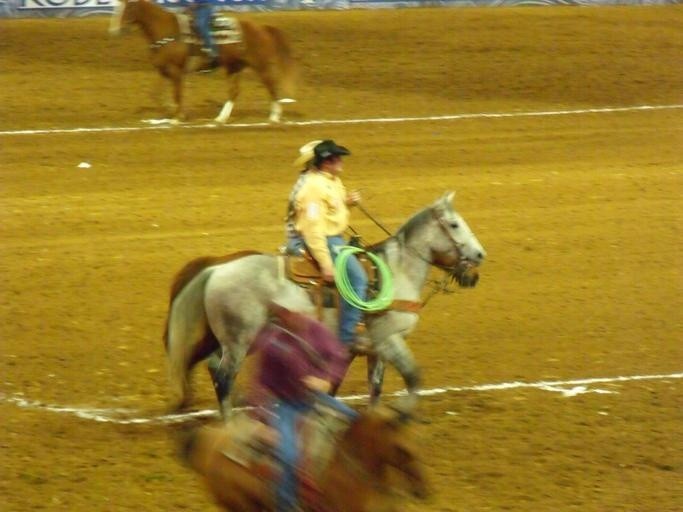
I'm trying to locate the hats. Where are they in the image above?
[294,140,350,167]
[270,282,315,313]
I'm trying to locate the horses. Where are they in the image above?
[157,190,488,422]
[110,1,296,128]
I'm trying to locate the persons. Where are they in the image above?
[284,140,373,356]
[175,1,226,74]
[237,278,350,512]
